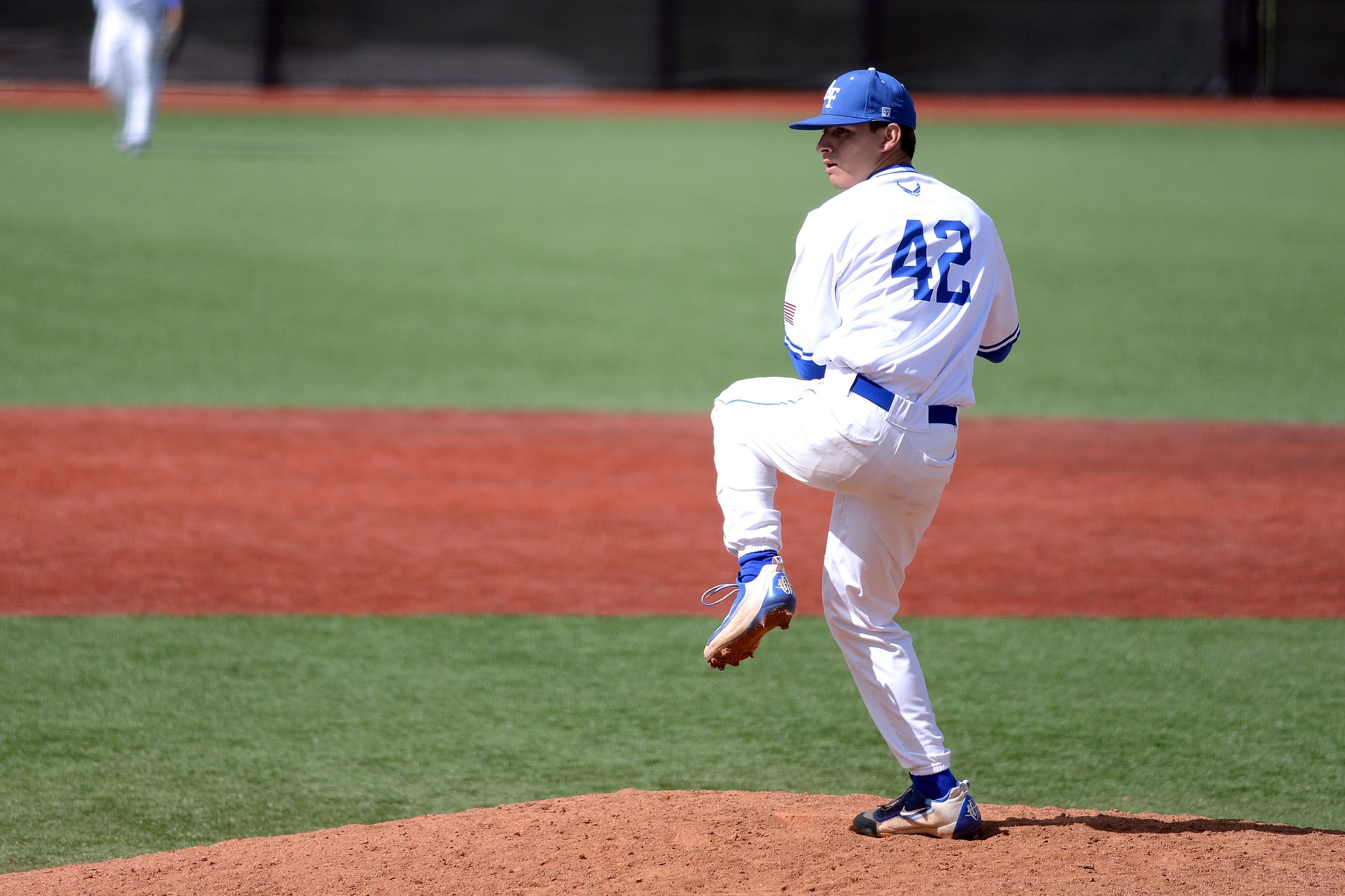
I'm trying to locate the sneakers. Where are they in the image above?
[701,555,797,673]
[852,777,985,839]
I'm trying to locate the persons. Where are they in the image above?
[703,68,1022,842]
[89,0,181,148]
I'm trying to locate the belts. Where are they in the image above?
[852,373,961,428]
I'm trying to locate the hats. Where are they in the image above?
[787,67,920,133]
